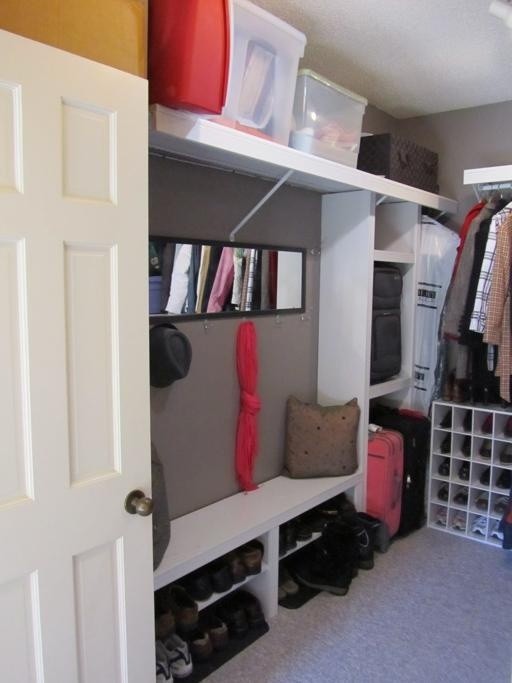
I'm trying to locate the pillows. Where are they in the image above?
[283,395,360,478]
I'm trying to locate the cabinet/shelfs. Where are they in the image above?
[153,475,364,622]
[428,399,512,549]
[317,191,421,513]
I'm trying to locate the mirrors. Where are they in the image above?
[149,235,306,323]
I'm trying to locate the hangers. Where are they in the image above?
[478,183,504,203]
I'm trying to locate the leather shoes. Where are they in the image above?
[442,380,490,405]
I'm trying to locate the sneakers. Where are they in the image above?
[436,408,512,541]
[157,540,264,683]
[279,493,381,600]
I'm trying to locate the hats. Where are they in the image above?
[149,323,191,388]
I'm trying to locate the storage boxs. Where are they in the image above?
[209,0,307,147]
[289,69,368,169]
[358,133,439,193]
[0,0,149,78]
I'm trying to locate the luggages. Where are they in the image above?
[371,266,403,384]
[369,404,431,536]
